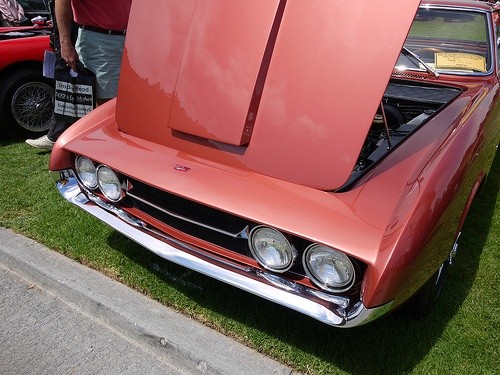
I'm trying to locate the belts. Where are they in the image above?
[79,24,126,36]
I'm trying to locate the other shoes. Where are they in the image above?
[25,134,55,153]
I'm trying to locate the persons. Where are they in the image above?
[25,0,72,150]
[54,1,132,106]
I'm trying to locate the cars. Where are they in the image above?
[0,0,88,135]
[47,0,499,328]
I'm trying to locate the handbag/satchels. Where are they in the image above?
[53,56,98,123]
